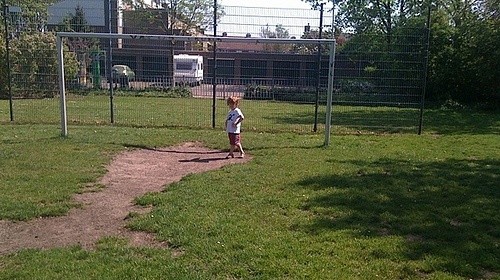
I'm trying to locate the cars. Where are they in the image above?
[107,65,135,84]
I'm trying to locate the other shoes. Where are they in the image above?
[238,152,245,158]
[227,154,234,158]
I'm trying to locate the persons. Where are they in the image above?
[225,96,245,159]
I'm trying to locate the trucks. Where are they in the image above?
[172,53,204,87]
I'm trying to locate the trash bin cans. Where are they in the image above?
[119,75,129,88]
